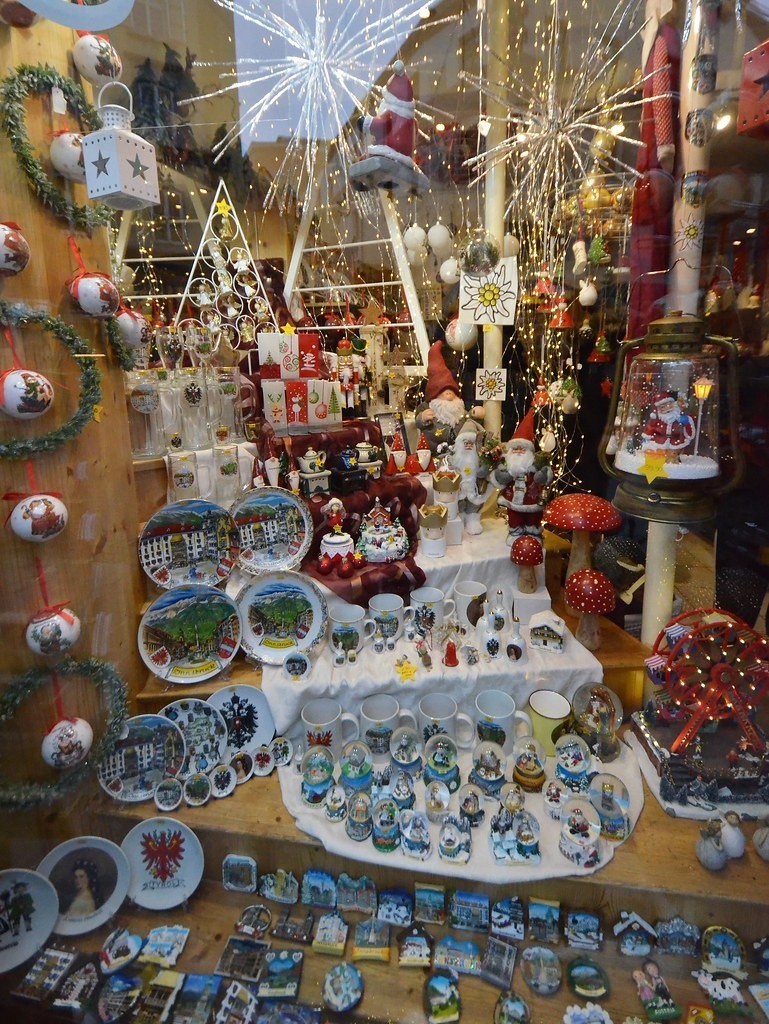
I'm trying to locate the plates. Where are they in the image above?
[0,868,59,974]
[282,652,311,679]
[138,498,241,590]
[119,816,205,911]
[226,486,313,575]
[566,957,609,997]
[36,835,131,935]
[233,569,328,664]
[519,944,562,993]
[93,683,294,810]
[137,584,243,683]
[322,961,364,1012]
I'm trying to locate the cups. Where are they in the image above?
[327,604,376,655]
[410,587,456,635]
[368,593,415,643]
[123,325,256,510]
[454,581,487,627]
[300,689,575,757]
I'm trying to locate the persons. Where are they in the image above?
[255,302,270,322]
[325,498,344,537]
[489,429,554,547]
[237,275,257,297]
[222,296,238,318]
[632,957,675,1009]
[211,245,227,270]
[222,327,235,350]
[185,321,201,345]
[416,374,469,455]
[218,274,233,297]
[437,418,494,535]
[196,283,212,306]
[233,252,250,275]
[642,395,695,464]
[206,311,220,335]
[239,317,253,344]
[218,216,233,240]
[66,859,102,916]
[351,61,419,193]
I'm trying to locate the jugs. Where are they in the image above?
[333,440,378,471]
[296,446,327,474]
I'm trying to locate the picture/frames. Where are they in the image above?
[374,411,412,463]
